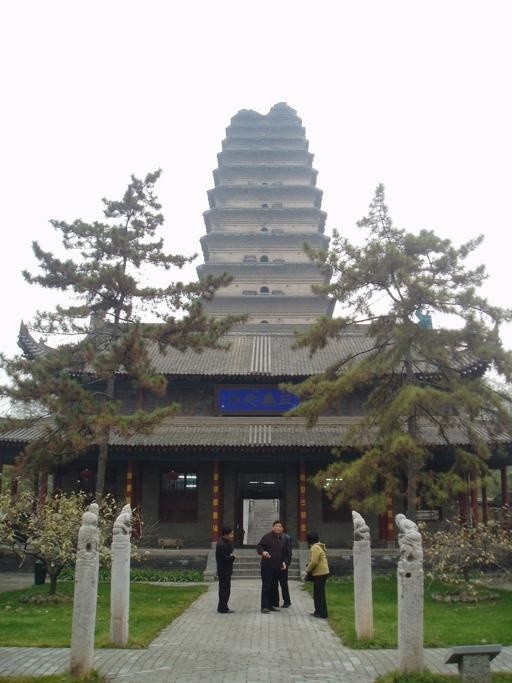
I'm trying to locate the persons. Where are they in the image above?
[300,530,330,618]
[214,525,240,612]
[274,531,291,609]
[256,519,286,613]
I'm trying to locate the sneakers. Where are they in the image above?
[282,603,291,608]
[269,606,280,612]
[221,609,234,613]
[310,611,322,618]
[261,608,268,613]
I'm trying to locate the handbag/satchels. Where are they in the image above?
[305,566,313,582]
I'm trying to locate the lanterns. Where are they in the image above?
[164,469,179,481]
[79,467,96,480]
